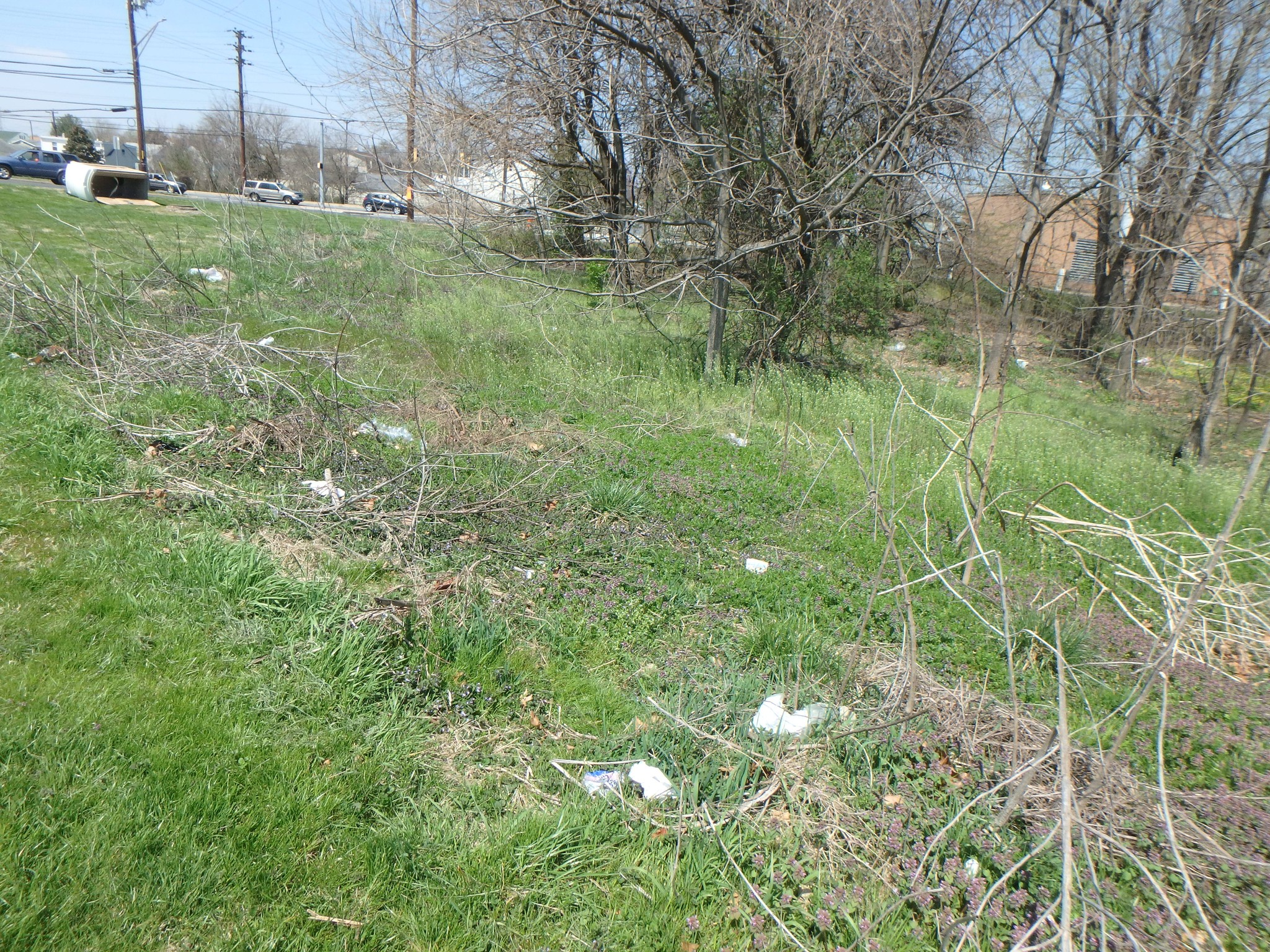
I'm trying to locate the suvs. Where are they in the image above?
[243,180,304,205]
[0,150,85,186]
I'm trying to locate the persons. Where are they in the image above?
[29,153,40,161]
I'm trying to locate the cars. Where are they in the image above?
[363,193,408,215]
[148,172,187,194]
